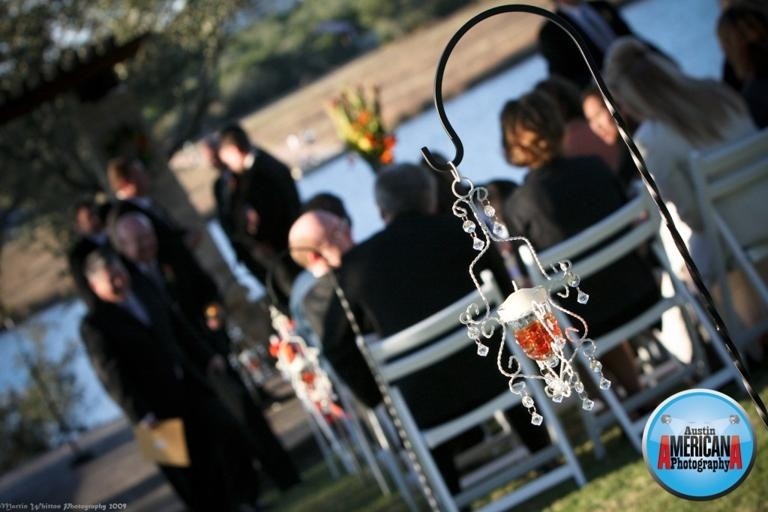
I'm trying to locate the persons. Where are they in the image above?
[66,1,768,509]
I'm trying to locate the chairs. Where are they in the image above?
[287,132,768,512]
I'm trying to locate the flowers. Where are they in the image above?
[327,80,395,172]
